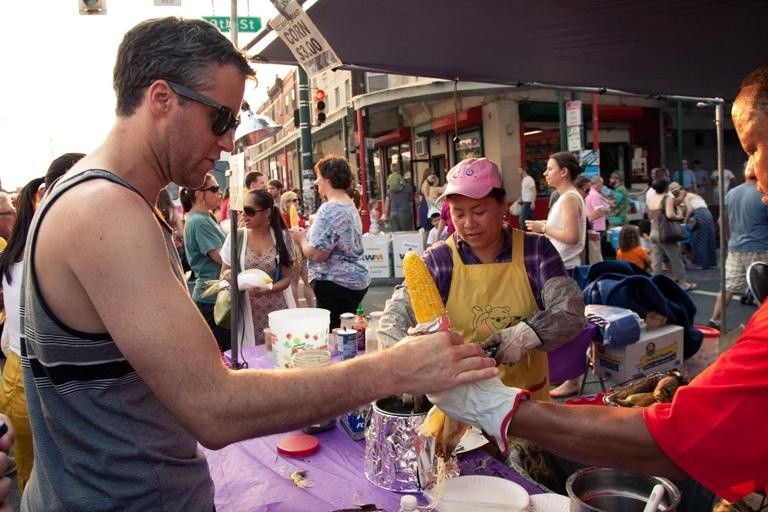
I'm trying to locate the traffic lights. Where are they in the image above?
[312,90,326,125]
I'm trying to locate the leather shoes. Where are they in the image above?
[548,385,580,397]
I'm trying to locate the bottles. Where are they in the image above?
[338,329,358,361]
[352,303,367,350]
[338,312,356,331]
[365,311,384,354]
[290,349,337,434]
[398,495,419,511]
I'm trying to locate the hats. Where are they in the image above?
[436,157,504,203]
[668,182,682,193]
[611,170,624,181]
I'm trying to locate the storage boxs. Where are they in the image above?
[590,322,684,386]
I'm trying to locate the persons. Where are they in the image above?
[0,153,103,505]
[221,188,299,347]
[405,68,767,511]
[290,156,374,326]
[523,153,592,395]
[2,148,766,355]
[182,168,234,353]
[18,16,500,511]
[378,157,588,405]
[0,413,18,512]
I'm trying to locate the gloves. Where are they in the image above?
[425,375,532,456]
[485,322,544,367]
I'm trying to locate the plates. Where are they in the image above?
[526,492,570,512]
[431,475,529,511]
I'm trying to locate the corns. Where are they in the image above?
[402,250,448,328]
[262,275,273,285]
[289,203,298,228]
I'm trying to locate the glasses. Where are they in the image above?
[244,208,262,216]
[291,199,298,202]
[585,189,589,193]
[0,210,17,215]
[201,186,219,193]
[150,80,239,136]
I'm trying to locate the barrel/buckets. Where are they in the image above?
[691,325,721,368]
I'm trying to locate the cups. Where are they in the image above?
[268,308,331,367]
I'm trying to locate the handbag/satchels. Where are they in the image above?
[658,211,687,242]
[687,217,697,231]
[213,279,243,328]
[580,229,603,265]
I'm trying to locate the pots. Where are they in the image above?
[566,467,681,512]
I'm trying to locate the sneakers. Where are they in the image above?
[681,284,697,291]
[688,264,703,269]
[711,319,723,327]
